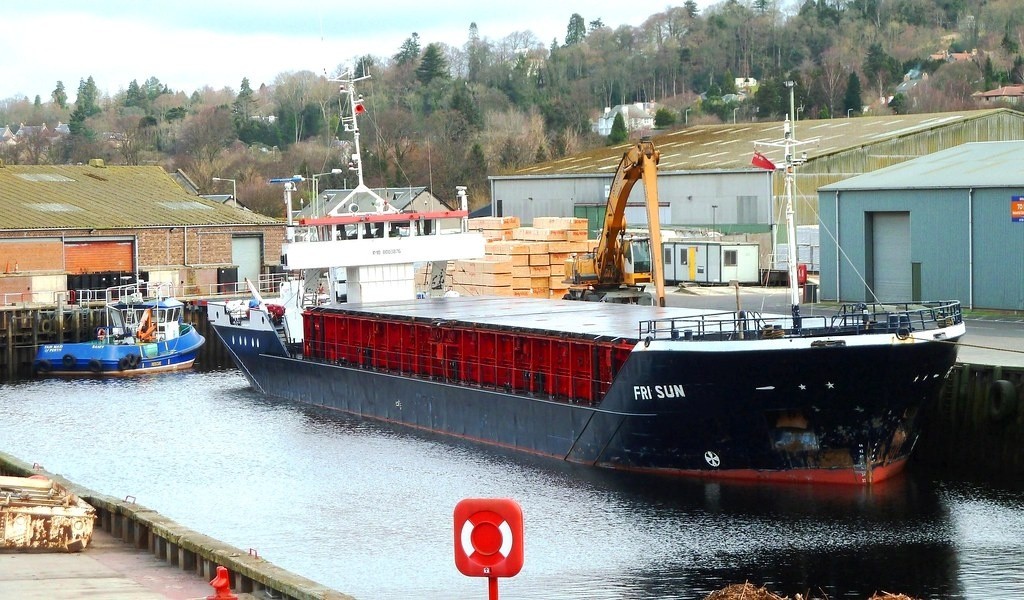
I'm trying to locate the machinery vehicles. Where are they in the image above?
[560,139,668,309]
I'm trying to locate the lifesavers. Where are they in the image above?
[98,328,106,340]
[63,353,76,367]
[119,354,141,370]
[987,379,1018,423]
[810,339,846,346]
[38,360,51,372]
[41,319,52,333]
[69,289,76,302]
[89,360,101,372]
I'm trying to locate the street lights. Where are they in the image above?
[797,107,800,122]
[686,109,691,124]
[783,81,801,271]
[734,107,739,124]
[301,177,319,217]
[312,172,334,217]
[711,206,718,236]
[848,109,853,118]
[212,177,236,206]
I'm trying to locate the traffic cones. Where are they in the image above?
[12,263,21,274]
[3,261,10,274]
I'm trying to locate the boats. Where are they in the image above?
[245,304,286,323]
[32,280,206,376]
[205,55,966,482]
